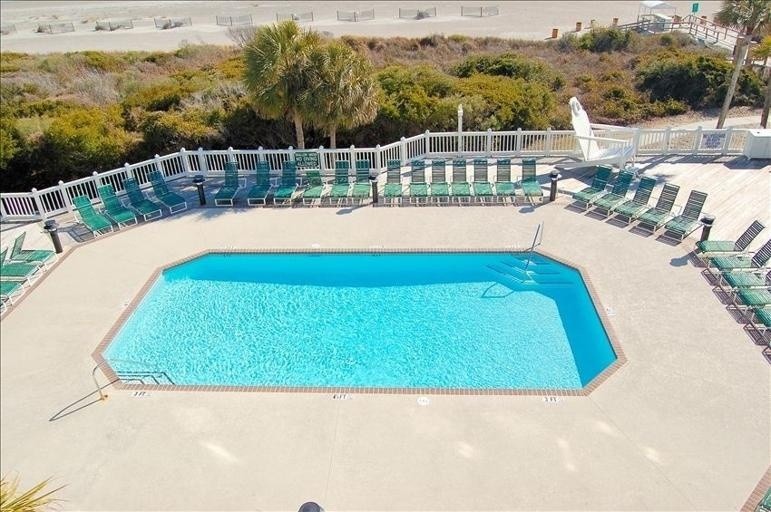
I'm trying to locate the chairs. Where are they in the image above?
[73,169,188,239]
[571,164,708,243]
[569,97,636,170]
[696,219,771,360]
[1,231,56,322]
[383,158,545,205]
[214,159,370,210]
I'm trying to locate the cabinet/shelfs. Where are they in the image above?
[743,129,771,163]
